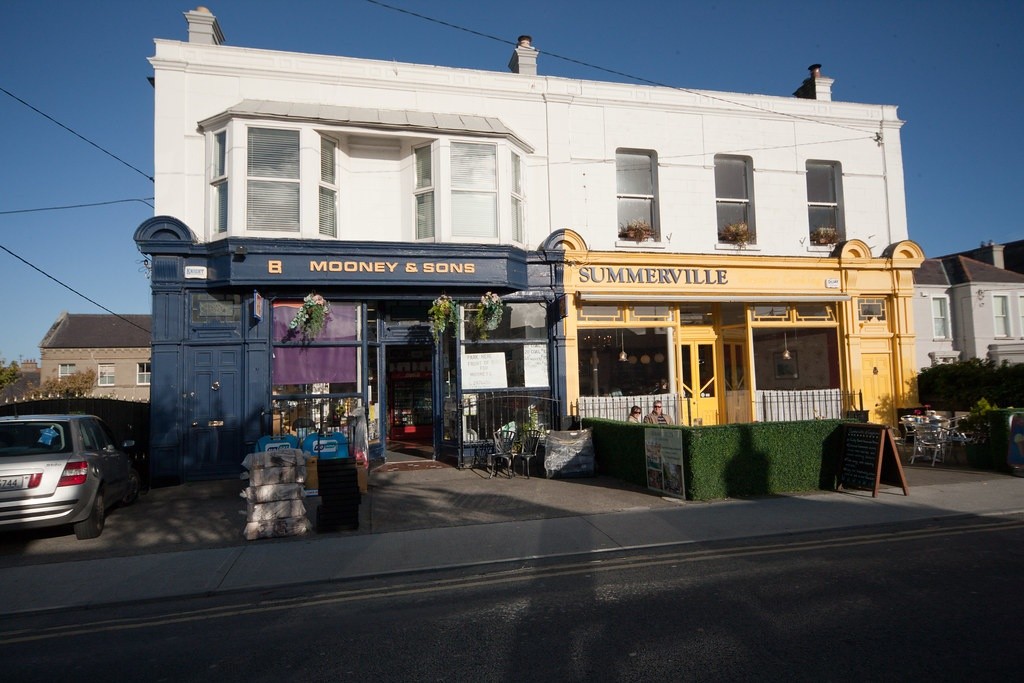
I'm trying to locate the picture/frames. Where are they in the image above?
[773,350,800,380]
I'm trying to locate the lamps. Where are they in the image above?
[235,246,247,255]
[782,329,792,360]
[619,329,628,361]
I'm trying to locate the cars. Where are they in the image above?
[0,413,140,540]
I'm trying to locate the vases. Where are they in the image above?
[726,237,737,241]
[819,237,835,244]
[307,306,315,315]
[625,230,651,239]
[443,307,450,316]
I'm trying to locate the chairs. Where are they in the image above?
[512,430,541,480]
[489,431,517,478]
[897,413,979,466]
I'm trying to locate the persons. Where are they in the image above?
[644,400,674,425]
[626,406,641,423]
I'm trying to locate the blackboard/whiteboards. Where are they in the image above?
[841,423,883,489]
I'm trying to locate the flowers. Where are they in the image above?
[288,292,331,340]
[428,294,461,346]
[811,226,839,242]
[472,293,504,342]
[718,222,756,250]
[620,219,656,242]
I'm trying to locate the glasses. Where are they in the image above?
[634,411,641,414]
[654,405,662,408]
